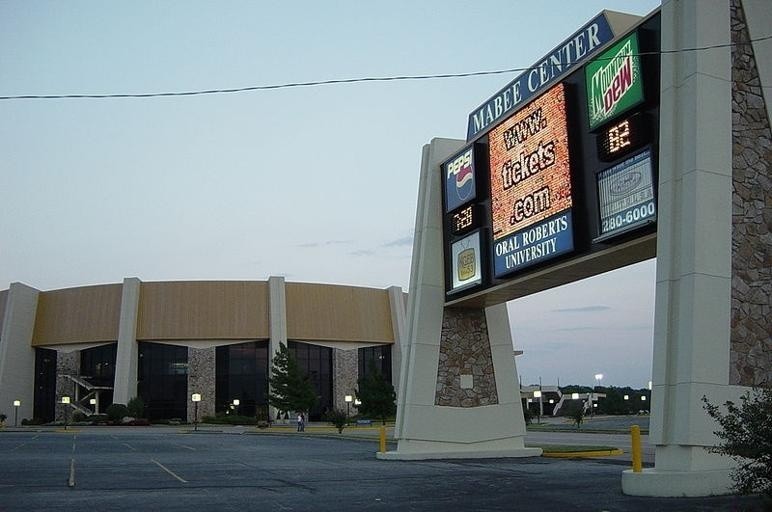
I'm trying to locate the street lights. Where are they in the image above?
[191,393,202,430]
[14,399,21,426]
[528,374,652,418]
[344,395,353,425]
[62,396,71,430]
[90,398,97,421]
[355,398,362,411]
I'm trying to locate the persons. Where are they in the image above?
[297,413,305,432]
